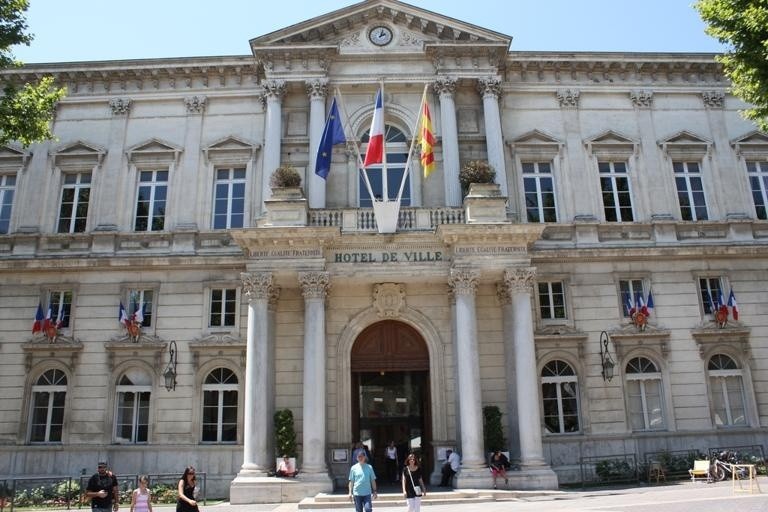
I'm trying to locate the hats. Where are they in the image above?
[356,451,366,457]
[97,459,108,468]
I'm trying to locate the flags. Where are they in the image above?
[625,283,739,321]
[30,290,144,336]
[315,98,347,181]
[364,91,383,169]
[417,91,438,179]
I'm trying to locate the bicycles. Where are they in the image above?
[709,449,757,483]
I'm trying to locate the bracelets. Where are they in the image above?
[114,502,119,505]
[373,489,377,491]
[372,491,377,493]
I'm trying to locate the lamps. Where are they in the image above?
[597,331,615,384]
[162,338,179,392]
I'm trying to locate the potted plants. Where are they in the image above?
[268,160,306,198]
[482,404,510,463]
[272,408,297,475]
[458,158,503,197]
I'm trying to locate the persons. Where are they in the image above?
[402,453,426,511]
[351,439,509,489]
[130,474,153,512]
[85,458,119,511]
[175,465,201,511]
[347,451,379,511]
[276,454,294,477]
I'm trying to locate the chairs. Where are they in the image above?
[688,460,714,486]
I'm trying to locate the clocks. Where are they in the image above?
[361,19,398,51]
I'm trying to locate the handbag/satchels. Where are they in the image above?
[412,484,423,497]
